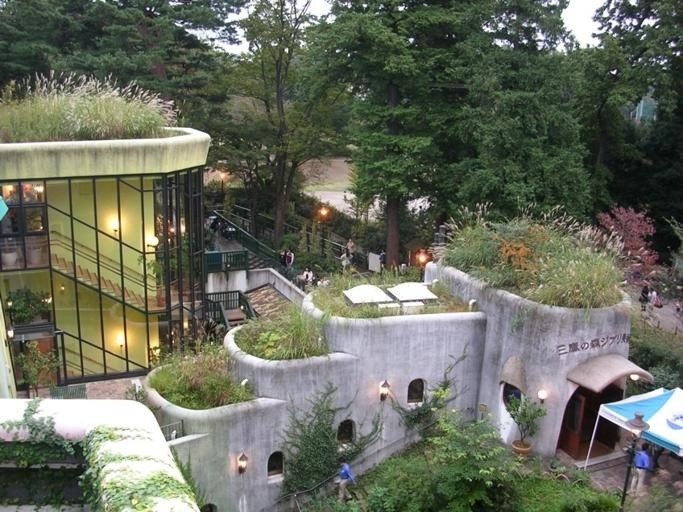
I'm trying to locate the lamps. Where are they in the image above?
[538,390,547,404]
[380,379,390,401]
[238,448,248,475]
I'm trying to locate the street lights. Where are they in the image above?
[416,251,430,280]
[319,204,329,254]
[620,409,650,511]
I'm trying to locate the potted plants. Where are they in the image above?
[505,394,550,458]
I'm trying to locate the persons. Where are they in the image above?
[317,276,328,286]
[336,454,358,506]
[345,238,355,260]
[628,441,650,495]
[379,249,386,269]
[279,249,286,265]
[303,266,312,287]
[644,286,657,316]
[638,280,649,312]
[286,249,294,267]
[334,248,354,271]
